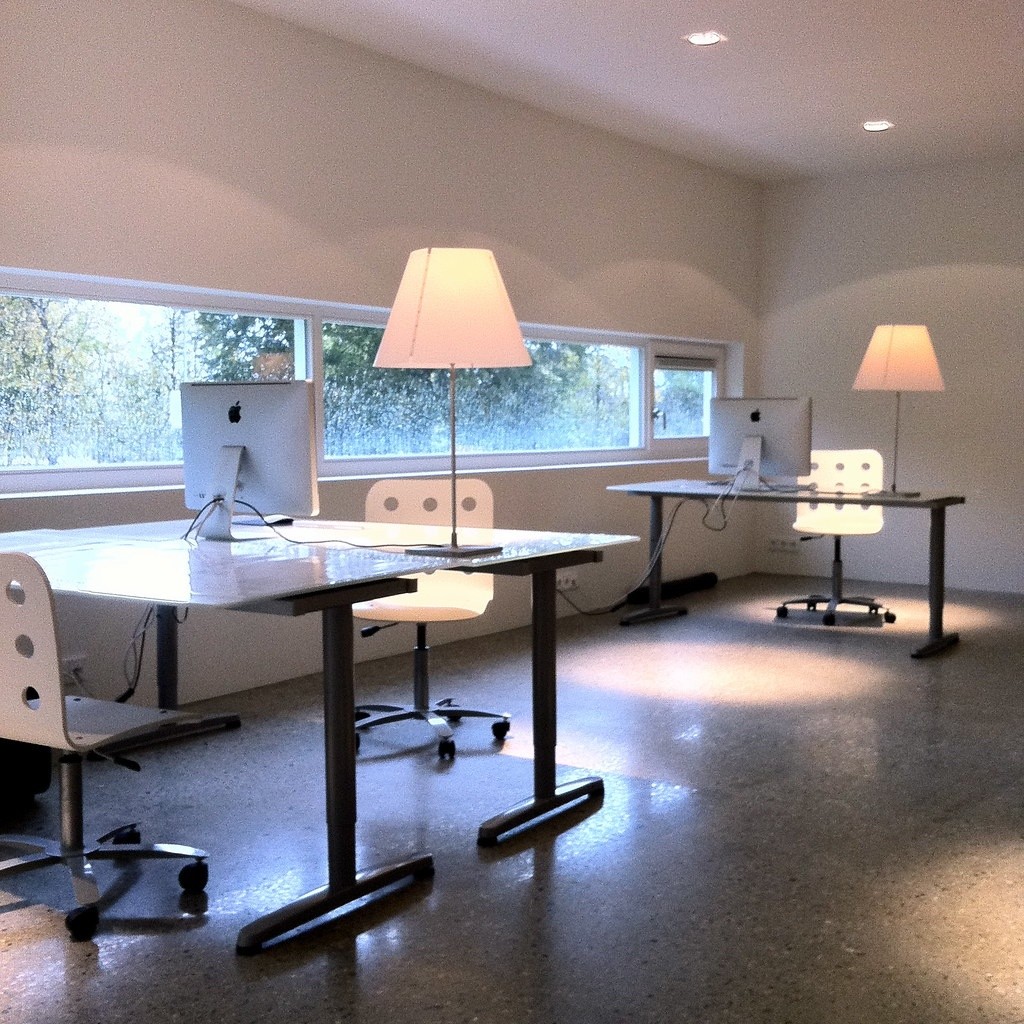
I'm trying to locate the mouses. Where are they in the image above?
[264,515,293,525]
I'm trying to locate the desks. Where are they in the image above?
[95,515,643,851]
[603,477,967,661]
[0,529,470,958]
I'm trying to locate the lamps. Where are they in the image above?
[847,325,948,498]
[368,240,532,561]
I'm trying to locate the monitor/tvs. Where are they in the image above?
[180,380,322,517]
[709,397,813,476]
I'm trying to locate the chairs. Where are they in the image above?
[339,477,513,762]
[0,551,240,941]
[775,450,898,625]
